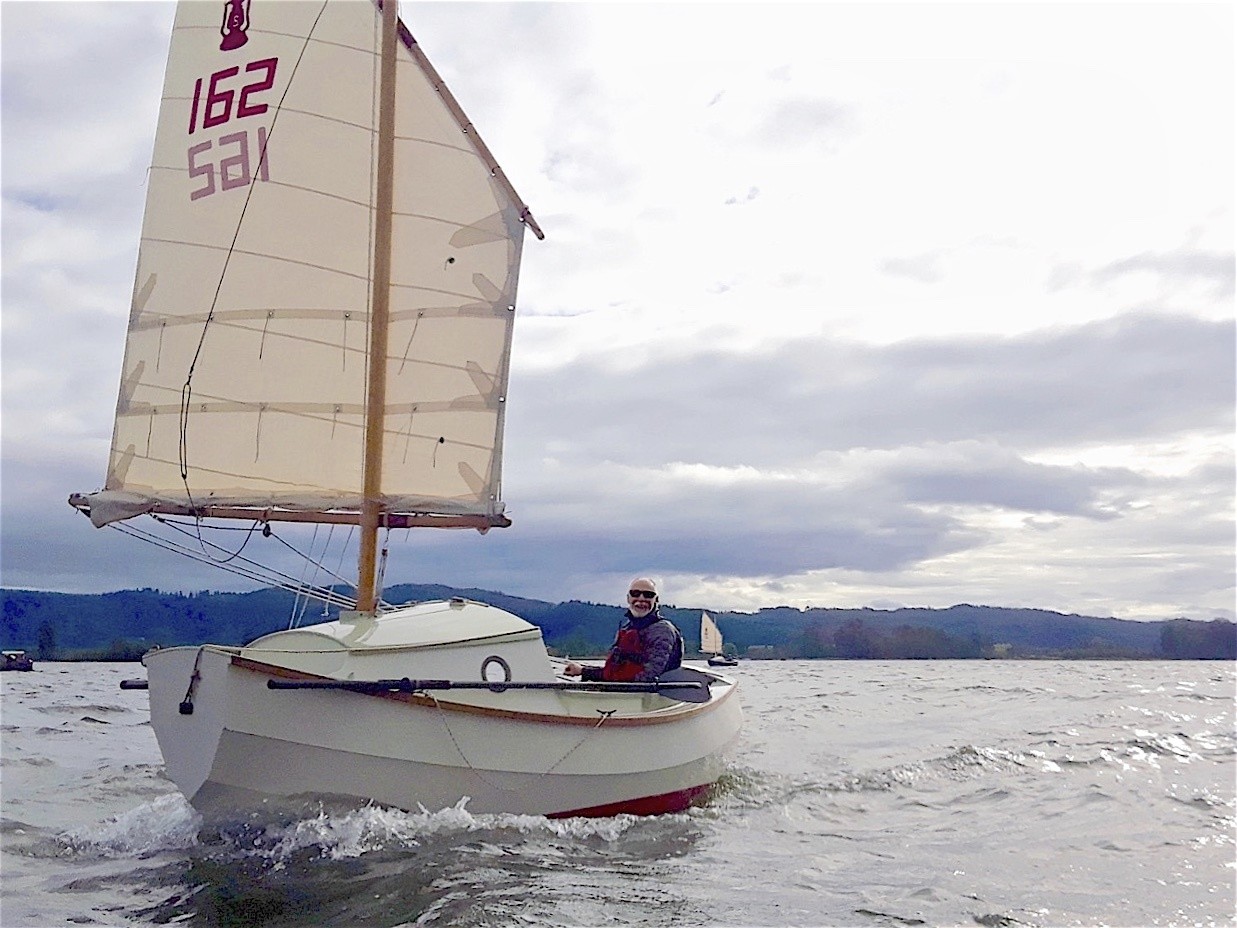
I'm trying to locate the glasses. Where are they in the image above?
[629,589,657,599]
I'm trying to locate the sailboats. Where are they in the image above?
[66,0,743,851]
[697,610,738,667]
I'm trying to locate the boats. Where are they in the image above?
[0,650,32,672]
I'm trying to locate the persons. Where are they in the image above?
[559,577,678,686]
